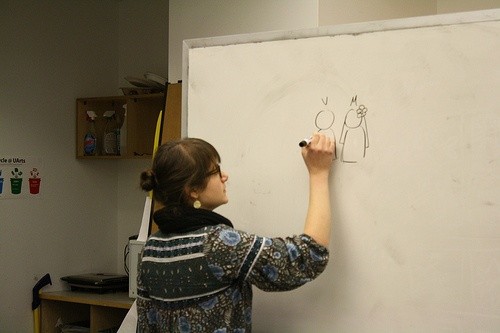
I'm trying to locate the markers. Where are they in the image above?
[299,138,314,148]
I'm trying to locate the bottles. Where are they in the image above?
[84,126,96,155]
[102,114,122,155]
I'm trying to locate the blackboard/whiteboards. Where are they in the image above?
[178,8,500,333]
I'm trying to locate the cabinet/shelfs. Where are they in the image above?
[74,95,161,161]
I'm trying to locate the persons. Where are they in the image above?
[135,132,336,333]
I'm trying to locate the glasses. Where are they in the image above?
[204,165,222,178]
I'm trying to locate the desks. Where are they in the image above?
[37,289,134,333]
